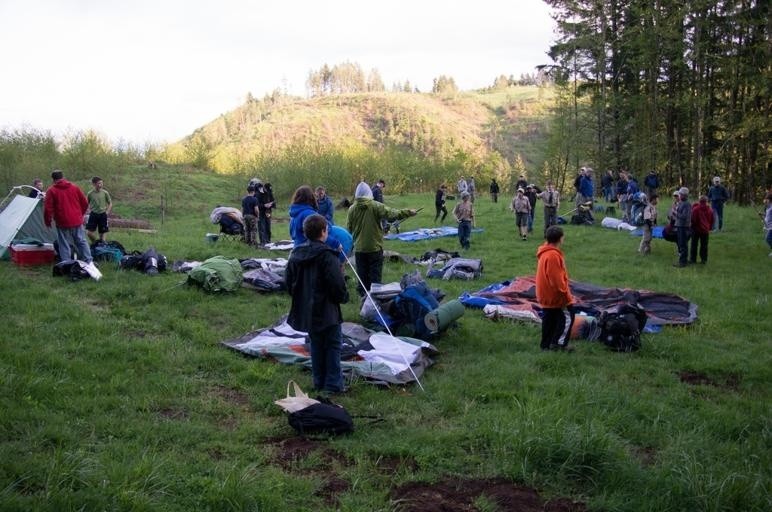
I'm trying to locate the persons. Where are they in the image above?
[645,170,660,197]
[468,177,475,203]
[457,176,468,199]
[668,190,680,228]
[241,187,260,244]
[581,168,594,217]
[289,186,342,254]
[510,187,531,240]
[517,174,527,190]
[255,182,272,243]
[370,179,389,233]
[532,181,560,237]
[348,181,419,297]
[452,193,477,251]
[638,195,659,255]
[284,215,352,396]
[616,171,626,209]
[535,226,578,351]
[761,193,772,257]
[708,176,729,231]
[689,194,716,265]
[526,184,542,231]
[214,204,245,235]
[43,169,93,267]
[257,184,276,247]
[85,176,112,242]
[672,187,693,268]
[27,178,44,198]
[601,170,616,203]
[314,186,334,226]
[489,178,500,203]
[434,184,448,224]
[574,166,587,213]
[625,174,640,223]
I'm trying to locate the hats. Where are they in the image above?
[680,188,689,196]
[713,177,720,182]
[461,191,469,198]
[517,188,524,194]
[586,168,594,173]
[673,192,679,195]
